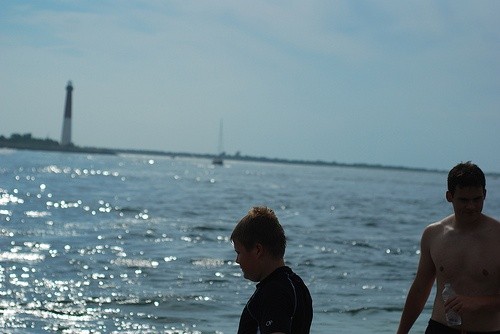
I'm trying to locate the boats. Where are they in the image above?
[211,158,224,165]
[0,133,118,154]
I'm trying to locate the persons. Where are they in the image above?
[397,162,500,334]
[229,207,313,334]
